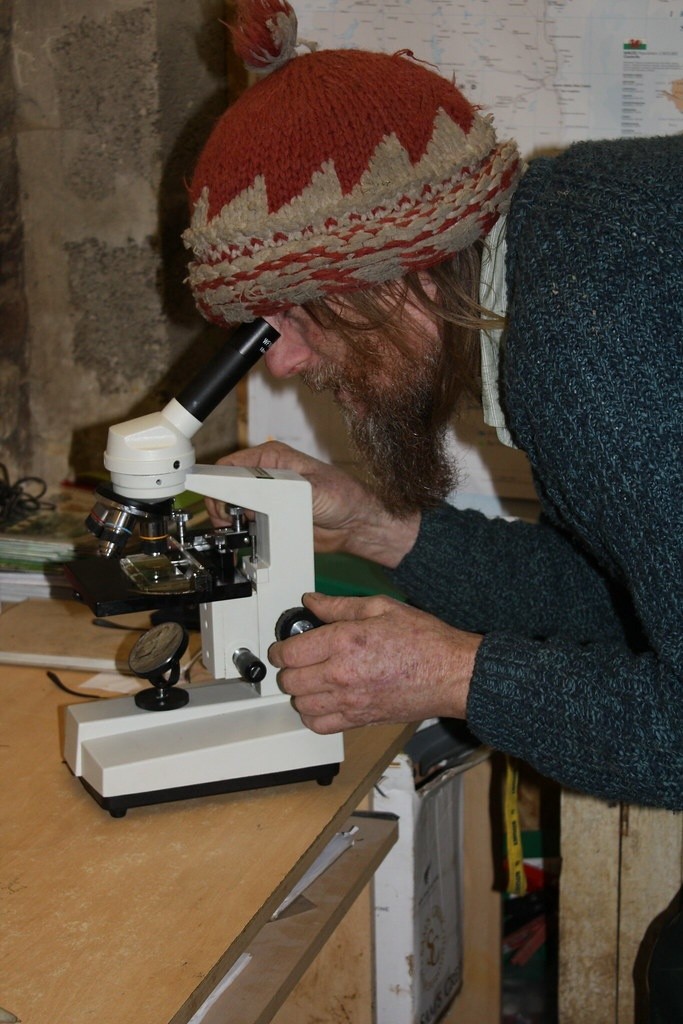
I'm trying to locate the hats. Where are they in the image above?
[186,0,527,330]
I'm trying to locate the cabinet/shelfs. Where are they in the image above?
[0,591,498,1024]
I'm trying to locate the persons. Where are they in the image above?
[181,0,683,1024]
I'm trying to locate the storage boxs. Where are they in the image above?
[373,717,464,1024]
